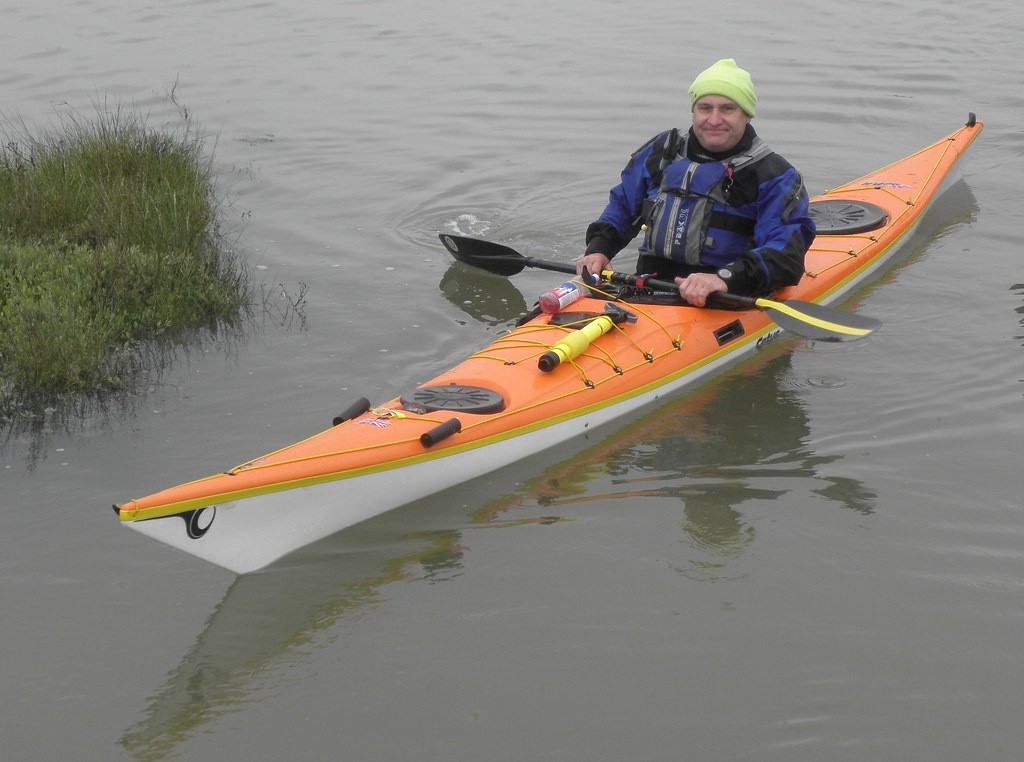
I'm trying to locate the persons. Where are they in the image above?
[576,58,816,310]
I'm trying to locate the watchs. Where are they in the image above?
[715,265,733,294]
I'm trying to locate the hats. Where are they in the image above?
[688,58,757,118]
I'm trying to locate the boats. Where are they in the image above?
[111,111,985,576]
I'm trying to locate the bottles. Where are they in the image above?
[539,274,599,314]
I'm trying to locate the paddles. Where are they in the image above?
[436,230,883,347]
